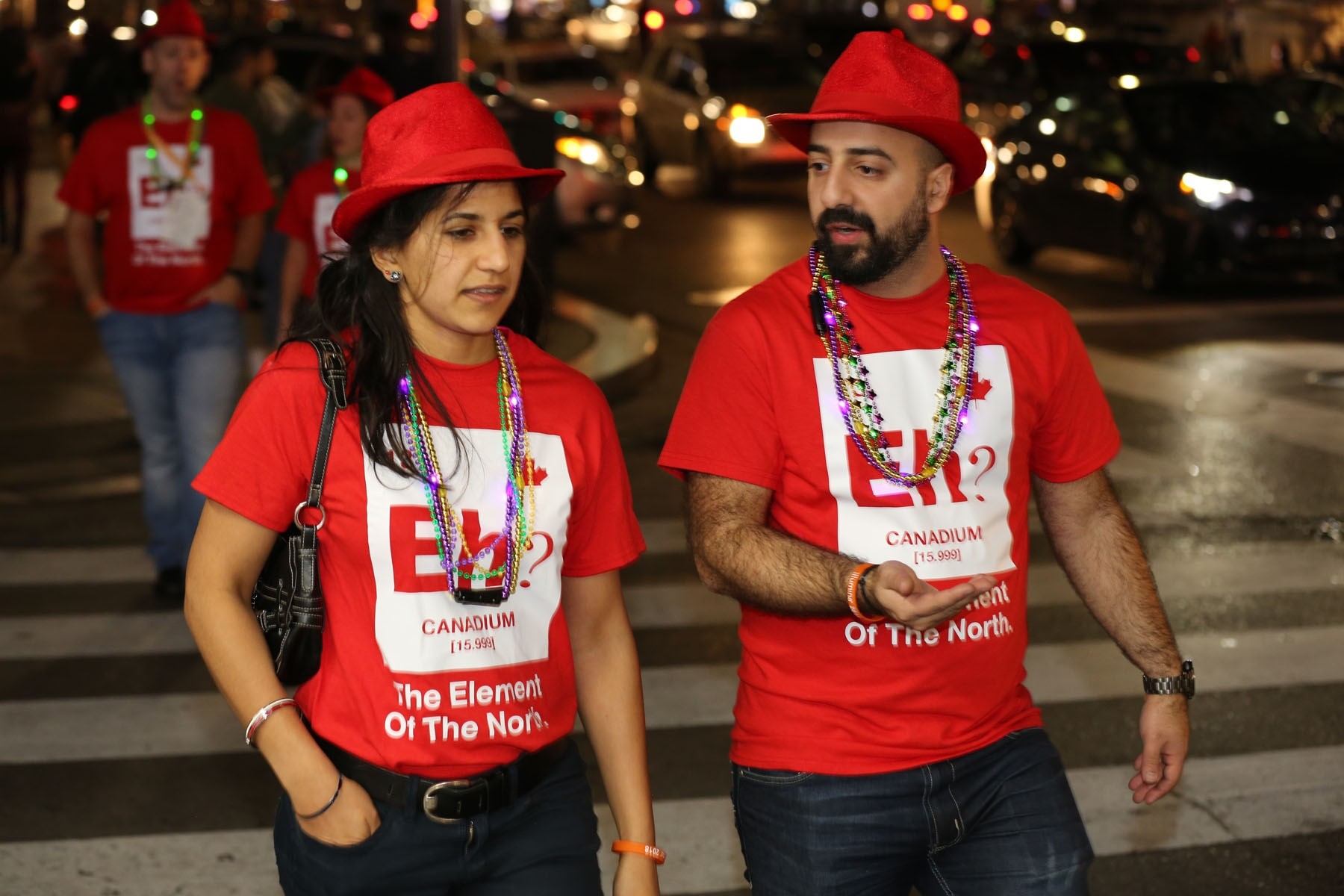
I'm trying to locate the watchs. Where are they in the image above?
[1139,659,1199,701]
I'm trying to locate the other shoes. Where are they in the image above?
[155,566,189,610]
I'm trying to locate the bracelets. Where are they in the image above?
[294,769,341,819]
[844,563,892,626]
[246,698,296,745]
[611,839,665,864]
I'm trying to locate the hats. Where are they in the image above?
[766,32,987,194]
[331,83,566,242]
[337,69,394,106]
[138,0,209,44]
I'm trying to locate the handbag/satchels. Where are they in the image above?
[246,328,347,689]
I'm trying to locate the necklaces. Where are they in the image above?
[137,91,202,192]
[806,241,979,482]
[401,326,532,607]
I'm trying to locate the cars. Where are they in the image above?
[984,72,1344,306]
[1264,70,1344,142]
[631,22,827,205]
[957,35,1194,146]
[482,42,660,194]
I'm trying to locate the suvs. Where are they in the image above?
[46,18,634,258]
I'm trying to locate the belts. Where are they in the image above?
[299,706,578,824]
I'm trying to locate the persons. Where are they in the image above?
[185,84,667,896]
[0,1,399,611]
[649,32,1192,896]
